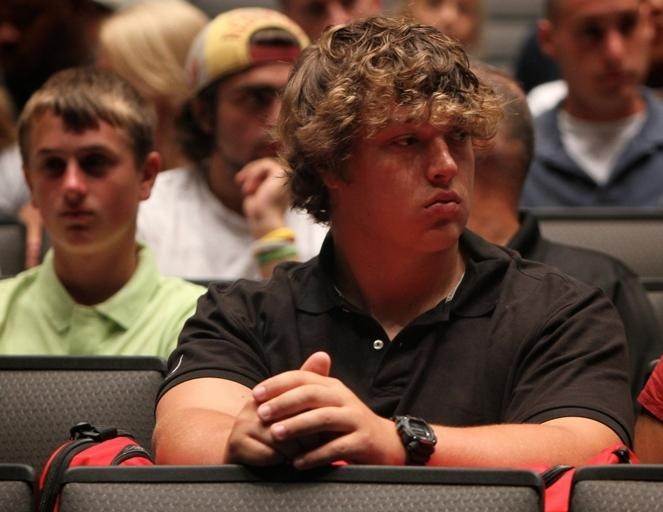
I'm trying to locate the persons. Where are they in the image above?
[462,60,662,355]
[149,15,636,476]
[632,351,663,464]
[0,66,209,363]
[0,1,662,278]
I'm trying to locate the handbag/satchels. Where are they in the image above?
[34,424,641,512]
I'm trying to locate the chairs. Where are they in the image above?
[0,207,663,512]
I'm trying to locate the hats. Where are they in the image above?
[179,8,313,107]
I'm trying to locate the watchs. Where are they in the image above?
[391,414,438,466]
